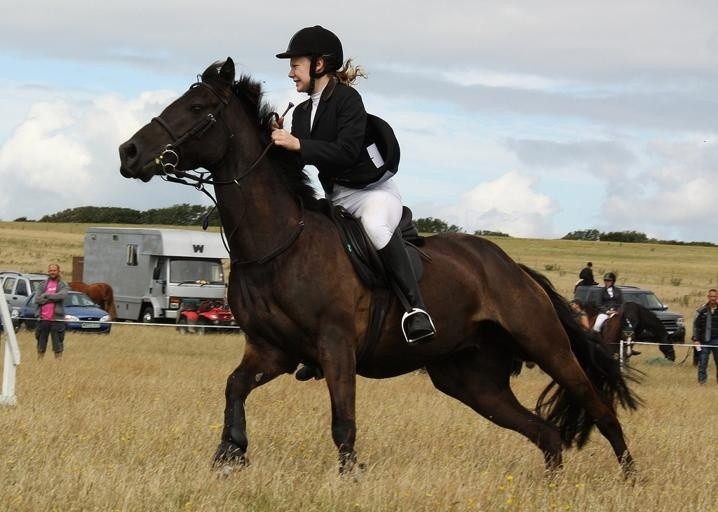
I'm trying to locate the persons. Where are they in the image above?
[691,289,718,383]
[35,264,68,360]
[570,262,641,355]
[271,25,433,381]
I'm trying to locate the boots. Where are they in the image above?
[382,235,434,340]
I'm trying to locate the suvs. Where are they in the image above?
[0,271,69,330]
[177,300,238,333]
[575,286,683,342]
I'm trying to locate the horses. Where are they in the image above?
[581,296,642,361]
[624,301,676,363]
[64,280,114,318]
[573,279,599,294]
[116,54,644,485]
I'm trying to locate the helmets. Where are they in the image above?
[275,24,344,71]
[604,272,616,281]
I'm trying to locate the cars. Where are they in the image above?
[24,289,112,334]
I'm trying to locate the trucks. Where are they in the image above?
[81,227,233,324]
